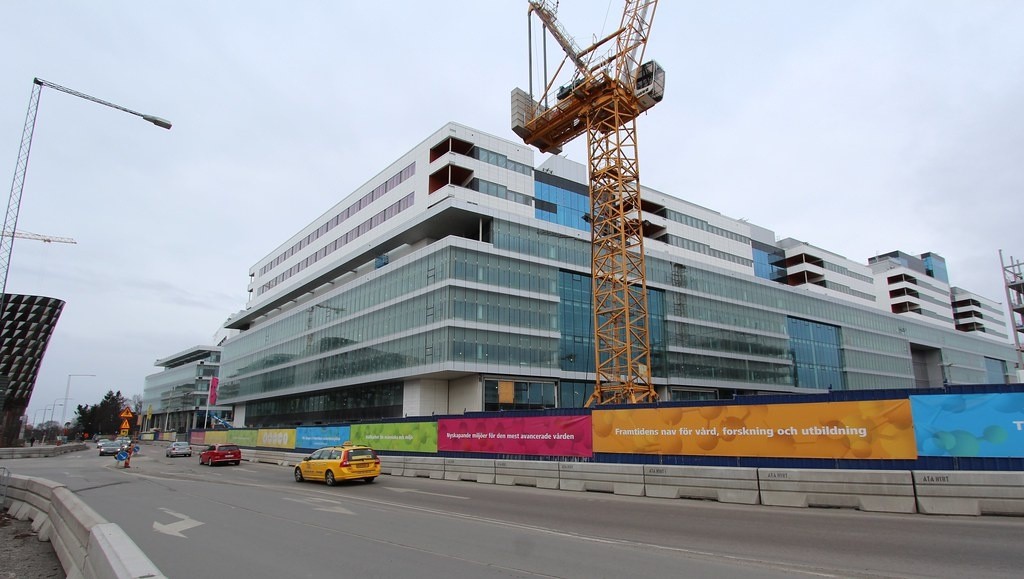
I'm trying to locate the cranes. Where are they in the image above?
[0,224,78,245]
[510,1,667,404]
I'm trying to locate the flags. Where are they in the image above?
[147,404,152,421]
[210,377,219,405]
[137,415,142,425]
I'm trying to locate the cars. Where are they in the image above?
[115,437,131,446]
[97,439,110,448]
[100,442,121,456]
[199,444,241,466]
[165,441,192,458]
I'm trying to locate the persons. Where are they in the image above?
[30,437,35,447]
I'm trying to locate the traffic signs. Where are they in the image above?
[121,429,128,436]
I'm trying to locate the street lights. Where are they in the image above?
[51,398,72,424]
[42,404,63,426]
[32,409,51,426]
[59,374,96,436]
[0,76,173,309]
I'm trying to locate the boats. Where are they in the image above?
[293,441,380,486]
